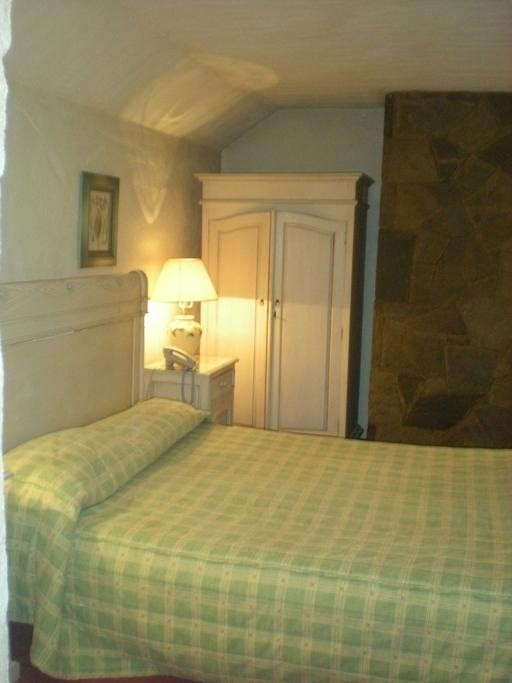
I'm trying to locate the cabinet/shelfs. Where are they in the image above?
[193,172,375,440]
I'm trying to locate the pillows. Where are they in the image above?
[3,397,210,510]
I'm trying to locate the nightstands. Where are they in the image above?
[144,357,239,425]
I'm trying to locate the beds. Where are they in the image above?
[1,270,512,683]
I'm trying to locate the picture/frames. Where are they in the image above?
[80,170,120,268]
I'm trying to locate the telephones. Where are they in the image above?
[163,346,195,369]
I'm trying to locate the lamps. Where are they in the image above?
[151,256,219,357]
